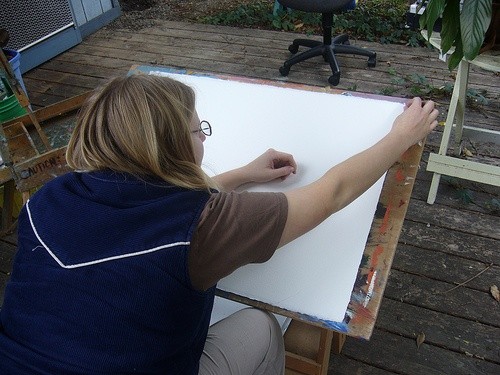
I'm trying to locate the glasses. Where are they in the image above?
[191,120,212,137]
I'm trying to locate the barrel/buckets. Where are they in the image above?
[0,90,27,122]
[0,48,33,112]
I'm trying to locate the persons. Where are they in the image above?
[0,73,439,375]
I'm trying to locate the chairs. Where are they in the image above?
[279,0,378,85]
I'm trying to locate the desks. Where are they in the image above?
[421,29,500,206]
